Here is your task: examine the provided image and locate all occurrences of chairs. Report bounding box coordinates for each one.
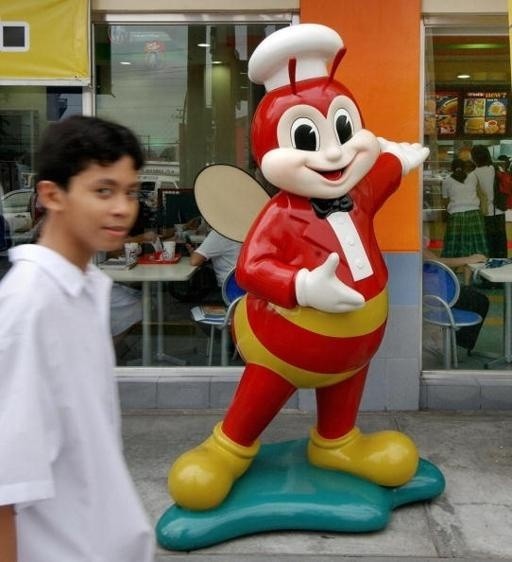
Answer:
[191,266,247,366]
[422,258,482,370]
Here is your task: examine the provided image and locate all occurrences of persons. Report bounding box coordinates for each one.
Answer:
[421,145,512,368]
[0,112,156,562]
[0,168,245,366]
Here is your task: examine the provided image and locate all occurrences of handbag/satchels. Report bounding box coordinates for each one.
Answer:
[494,169,512,211]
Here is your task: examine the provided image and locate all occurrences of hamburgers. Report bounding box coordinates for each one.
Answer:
[467,118,484,134]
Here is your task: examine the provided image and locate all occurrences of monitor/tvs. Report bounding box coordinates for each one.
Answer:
[157,187,202,235]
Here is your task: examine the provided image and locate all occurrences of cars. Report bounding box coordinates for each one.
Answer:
[0,159,181,247]
[422,162,451,207]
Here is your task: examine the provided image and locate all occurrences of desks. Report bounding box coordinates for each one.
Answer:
[99,233,203,367]
[466,257,512,370]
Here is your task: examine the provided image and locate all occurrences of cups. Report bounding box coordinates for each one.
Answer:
[124,242,138,265]
[163,241,176,259]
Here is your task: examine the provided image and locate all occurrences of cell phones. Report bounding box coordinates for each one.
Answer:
[185,235,192,247]
